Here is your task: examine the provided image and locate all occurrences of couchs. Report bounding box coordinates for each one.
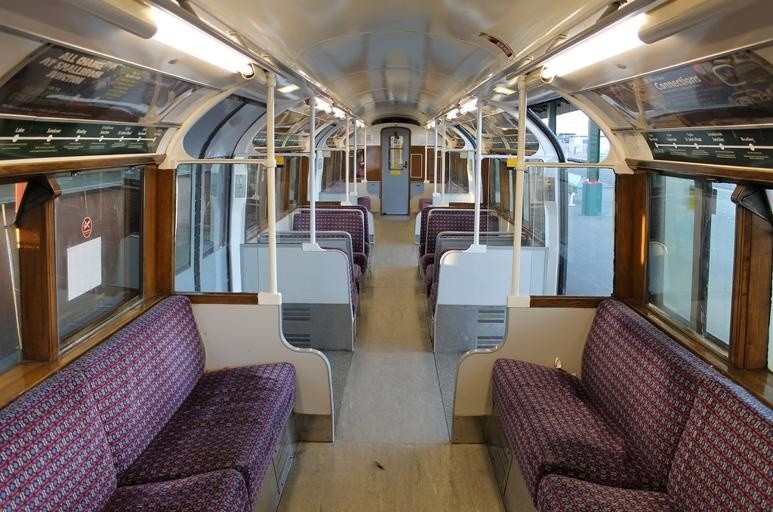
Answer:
[535,374,773,512]
[258,194,370,314]
[486,298,721,504]
[420,201,528,327]
[0,363,250,512]
[69,296,300,512]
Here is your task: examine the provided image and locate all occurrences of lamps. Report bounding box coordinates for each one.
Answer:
[539,7,653,82]
[146,6,253,80]
